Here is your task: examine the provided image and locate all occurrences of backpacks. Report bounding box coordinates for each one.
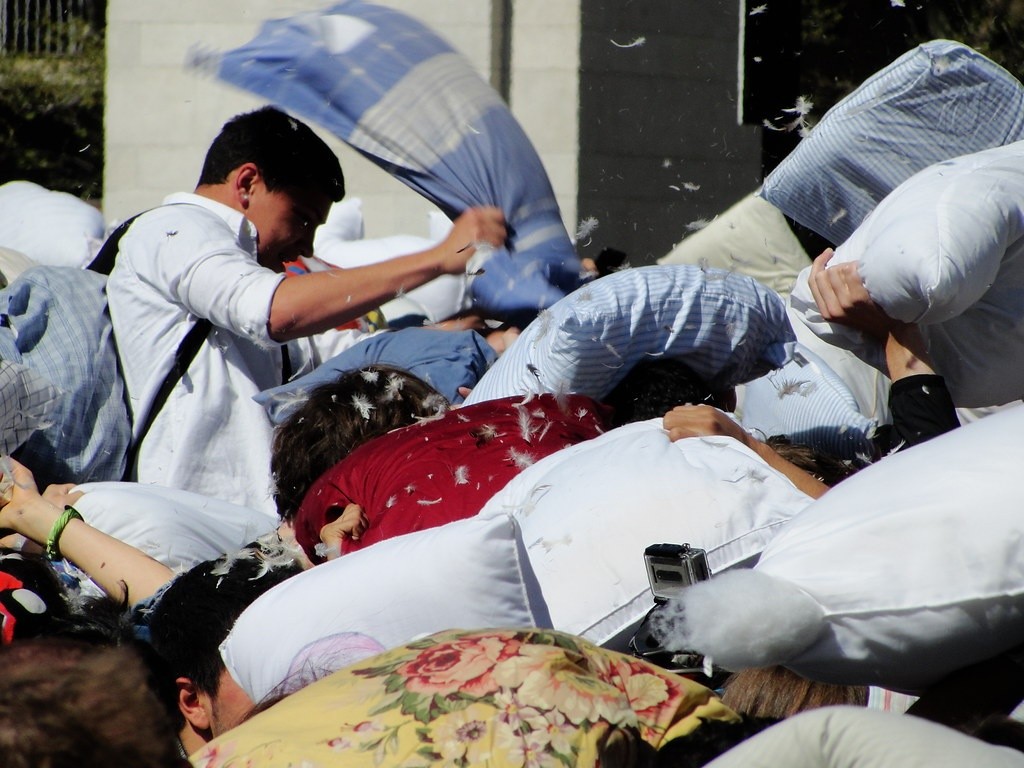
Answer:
[0,204,218,495]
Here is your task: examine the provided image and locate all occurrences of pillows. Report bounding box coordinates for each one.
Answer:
[0,265,134,495]
[743,341,879,461]
[251,327,500,431]
[187,628,743,768]
[0,359,72,456]
[652,402,1024,688]
[656,193,814,303]
[0,248,35,291]
[461,264,798,408]
[69,481,280,597]
[314,198,473,325]
[698,704,1024,768]
[755,39,1024,245]
[0,180,106,286]
[477,405,815,651]
[216,512,552,707]
[736,304,894,424]
[787,142,1024,407]
[185,0,587,312]
[293,393,613,566]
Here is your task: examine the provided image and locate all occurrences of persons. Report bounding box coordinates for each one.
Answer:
[2,206,1024,768]
[102,100,509,524]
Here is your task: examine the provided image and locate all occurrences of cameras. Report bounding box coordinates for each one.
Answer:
[627,543,713,678]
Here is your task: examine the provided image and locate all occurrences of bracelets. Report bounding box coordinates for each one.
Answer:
[46,503,85,561]
